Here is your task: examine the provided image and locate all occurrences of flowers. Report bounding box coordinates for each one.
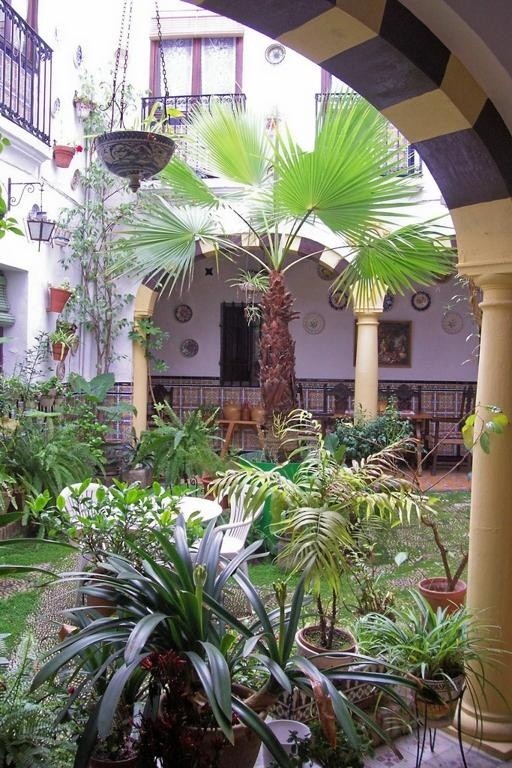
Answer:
[76,96,98,109]
[73,142,83,152]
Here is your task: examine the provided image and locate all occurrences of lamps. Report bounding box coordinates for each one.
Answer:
[7,176,55,252]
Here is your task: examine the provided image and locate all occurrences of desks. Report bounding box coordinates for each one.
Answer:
[121,494,223,541]
[300,413,433,477]
[216,418,265,459]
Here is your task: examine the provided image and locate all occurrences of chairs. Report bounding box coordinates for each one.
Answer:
[384,384,428,466]
[295,383,307,429]
[420,385,475,478]
[189,484,266,613]
[59,481,138,551]
[322,384,354,442]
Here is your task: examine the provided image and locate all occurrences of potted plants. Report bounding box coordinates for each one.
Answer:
[49,281,73,314]
[80,102,185,192]
[1,368,151,517]
[50,331,77,359]
[353,398,509,623]
[17,504,511,768]
[197,402,218,428]
[205,403,447,679]
[94,117,462,554]
[222,266,272,325]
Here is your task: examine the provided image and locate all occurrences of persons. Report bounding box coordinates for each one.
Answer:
[379,328,408,355]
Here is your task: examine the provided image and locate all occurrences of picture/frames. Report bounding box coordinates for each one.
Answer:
[350,317,414,370]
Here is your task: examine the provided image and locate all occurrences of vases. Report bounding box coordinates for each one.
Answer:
[252,400,268,424]
[204,477,228,507]
[241,401,251,421]
[53,145,73,167]
[76,107,91,118]
[221,398,241,421]
